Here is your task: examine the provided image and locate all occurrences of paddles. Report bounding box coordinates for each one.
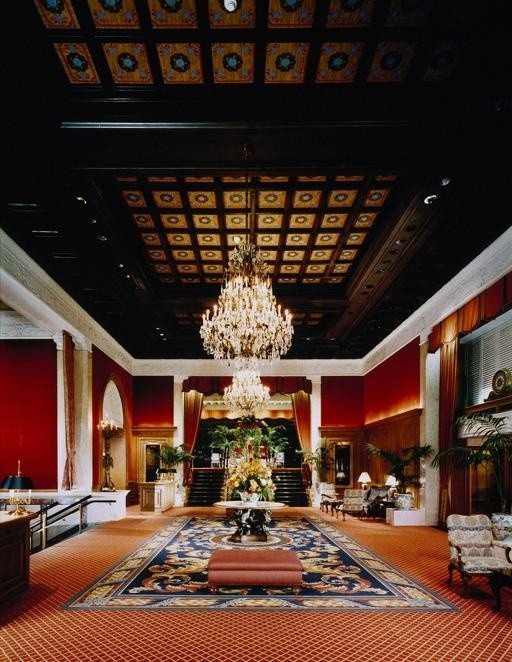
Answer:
[59,514,462,612]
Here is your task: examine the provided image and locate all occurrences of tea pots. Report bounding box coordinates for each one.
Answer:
[332,441,353,488]
[144,443,162,482]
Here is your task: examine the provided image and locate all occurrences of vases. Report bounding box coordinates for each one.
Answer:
[226,457,277,501]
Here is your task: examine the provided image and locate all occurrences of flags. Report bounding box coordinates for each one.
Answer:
[236,490,260,502]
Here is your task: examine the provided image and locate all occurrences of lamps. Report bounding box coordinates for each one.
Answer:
[446,513,512,610]
[318,482,399,521]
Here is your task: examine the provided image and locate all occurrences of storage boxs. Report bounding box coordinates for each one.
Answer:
[207,550,304,595]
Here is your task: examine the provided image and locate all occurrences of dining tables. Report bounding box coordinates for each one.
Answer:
[360,439,435,510]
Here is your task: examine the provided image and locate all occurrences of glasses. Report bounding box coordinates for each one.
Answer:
[97,413,118,492]
[336,472,346,481]
[224,369,270,420]
[1,476,36,517]
[358,471,371,490]
[156,468,177,483]
[384,475,400,499]
[198,239,296,368]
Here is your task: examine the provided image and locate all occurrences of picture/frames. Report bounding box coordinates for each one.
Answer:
[213,500,288,543]
